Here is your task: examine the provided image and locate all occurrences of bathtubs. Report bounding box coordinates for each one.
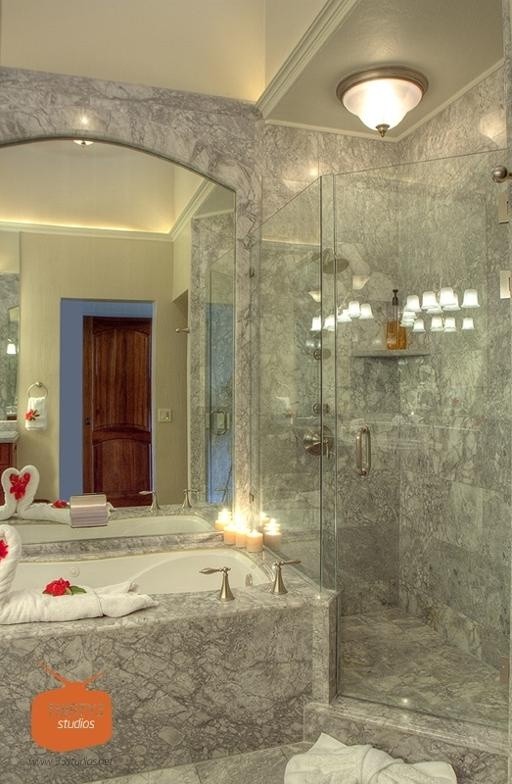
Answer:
[0,514,217,544]
[10,546,274,595]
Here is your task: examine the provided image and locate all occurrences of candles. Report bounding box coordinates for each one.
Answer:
[223,524,264,553]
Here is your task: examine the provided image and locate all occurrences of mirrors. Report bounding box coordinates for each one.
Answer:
[0,135,236,546]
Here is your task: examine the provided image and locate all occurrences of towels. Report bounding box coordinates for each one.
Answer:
[0,523,159,626]
[282,733,458,784]
[70,493,109,530]
[24,396,48,430]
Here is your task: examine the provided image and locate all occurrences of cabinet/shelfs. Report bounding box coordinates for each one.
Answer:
[0,442,19,507]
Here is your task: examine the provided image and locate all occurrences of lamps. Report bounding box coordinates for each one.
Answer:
[311,286,481,340]
[337,67,428,139]
